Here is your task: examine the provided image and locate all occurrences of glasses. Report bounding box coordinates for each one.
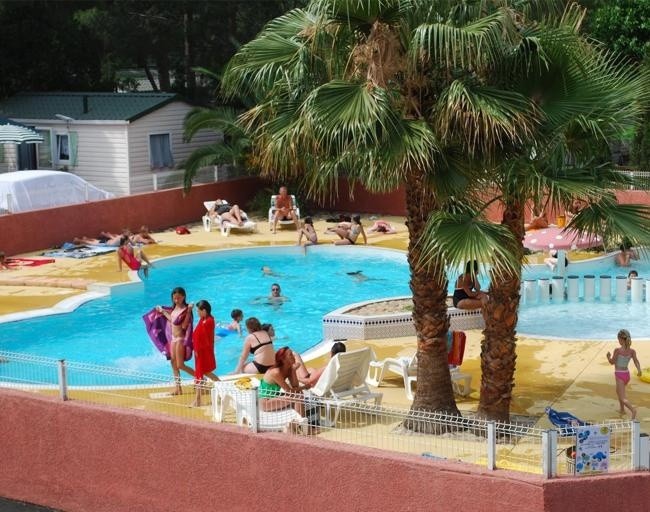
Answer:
[272,289,278,291]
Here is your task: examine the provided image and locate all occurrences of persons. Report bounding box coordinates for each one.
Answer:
[210,199,244,228]
[141,284,353,436]
[452,259,496,328]
[615,239,639,296]
[272,186,299,233]
[545,242,569,274]
[326,213,391,245]
[602,328,644,423]
[296,216,317,250]
[69,225,154,271]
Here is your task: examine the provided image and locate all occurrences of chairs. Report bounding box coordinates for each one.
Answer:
[210,327,472,434]
[202,200,257,239]
[267,195,300,232]
